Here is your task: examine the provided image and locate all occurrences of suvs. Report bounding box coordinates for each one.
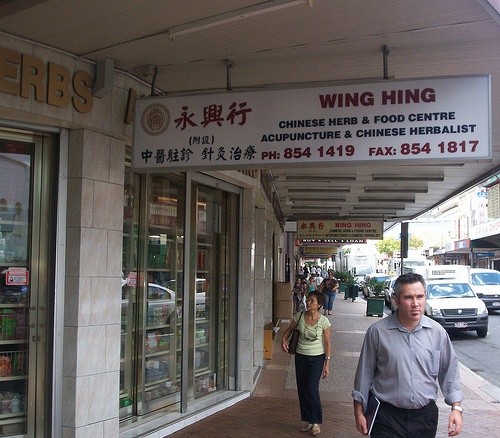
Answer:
[384,276,399,310]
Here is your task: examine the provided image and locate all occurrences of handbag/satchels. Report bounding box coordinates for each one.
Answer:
[289,310,305,354]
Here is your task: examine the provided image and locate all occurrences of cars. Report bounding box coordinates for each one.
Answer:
[162,278,207,314]
[121,280,177,327]
[363,274,391,299]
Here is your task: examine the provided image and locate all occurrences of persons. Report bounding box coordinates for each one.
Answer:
[291,263,339,314]
[352,273,465,438]
[281,291,331,436]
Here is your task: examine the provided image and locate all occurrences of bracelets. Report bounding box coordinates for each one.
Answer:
[324,356,331,360]
[452,406,464,414]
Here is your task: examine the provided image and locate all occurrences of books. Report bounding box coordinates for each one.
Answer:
[364,391,381,437]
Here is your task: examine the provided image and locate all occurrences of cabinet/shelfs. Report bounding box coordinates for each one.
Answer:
[120,218,228,399]
[0,203,28,426]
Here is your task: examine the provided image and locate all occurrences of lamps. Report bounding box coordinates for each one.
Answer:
[283,173,445,217]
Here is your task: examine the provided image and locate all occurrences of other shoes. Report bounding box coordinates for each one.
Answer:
[328,311,332,315]
[312,424,321,436]
[324,312,327,315]
[300,422,310,432]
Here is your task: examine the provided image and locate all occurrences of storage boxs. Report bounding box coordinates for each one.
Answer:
[264,329,277,359]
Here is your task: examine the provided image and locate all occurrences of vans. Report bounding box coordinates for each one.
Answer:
[469,268,500,311]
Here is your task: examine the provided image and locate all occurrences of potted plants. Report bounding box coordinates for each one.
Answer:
[334,271,359,298]
[364,276,389,317]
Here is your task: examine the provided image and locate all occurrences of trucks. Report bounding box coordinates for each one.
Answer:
[335,244,377,291]
[392,250,426,276]
[402,265,488,339]
